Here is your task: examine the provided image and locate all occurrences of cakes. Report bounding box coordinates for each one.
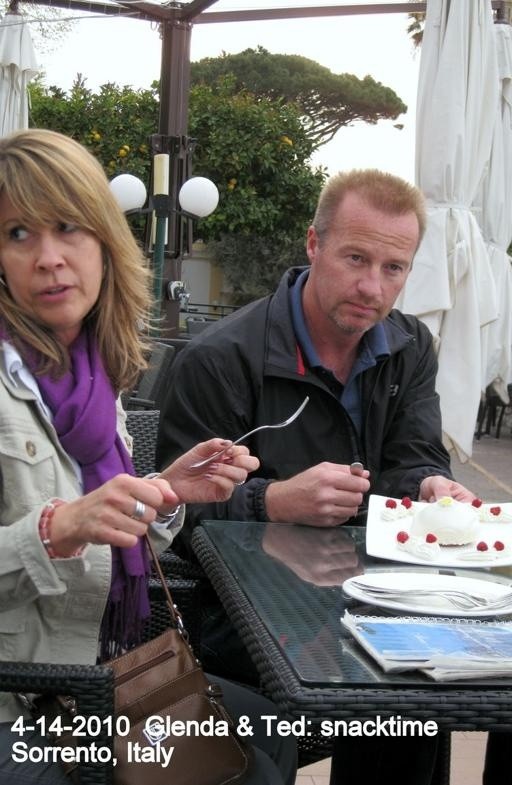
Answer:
[381,494,512,563]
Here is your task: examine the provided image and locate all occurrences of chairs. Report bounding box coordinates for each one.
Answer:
[129,314,230,407]
[474,382,512,440]
[125,408,206,578]
[0,574,206,785]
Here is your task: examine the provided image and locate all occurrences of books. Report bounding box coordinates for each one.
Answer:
[340,613,511,683]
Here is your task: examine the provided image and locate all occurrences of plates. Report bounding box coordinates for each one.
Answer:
[341,573,512,615]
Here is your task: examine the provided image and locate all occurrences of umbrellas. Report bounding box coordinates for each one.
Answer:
[0,14,40,138]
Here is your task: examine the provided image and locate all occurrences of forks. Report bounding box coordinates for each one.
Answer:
[188,395,310,470]
[350,580,512,611]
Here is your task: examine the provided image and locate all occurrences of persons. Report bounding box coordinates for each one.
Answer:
[0,129,298,785]
[155,169,512,785]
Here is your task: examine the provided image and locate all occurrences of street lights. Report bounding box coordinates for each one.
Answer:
[107,152,221,341]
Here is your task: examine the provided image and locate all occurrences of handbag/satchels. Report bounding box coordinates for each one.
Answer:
[30,626,254,785]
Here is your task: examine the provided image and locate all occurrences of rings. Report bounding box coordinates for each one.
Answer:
[130,499,146,521]
[233,480,246,486]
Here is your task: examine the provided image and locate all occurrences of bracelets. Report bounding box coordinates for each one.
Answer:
[143,472,181,524]
[39,499,87,558]
